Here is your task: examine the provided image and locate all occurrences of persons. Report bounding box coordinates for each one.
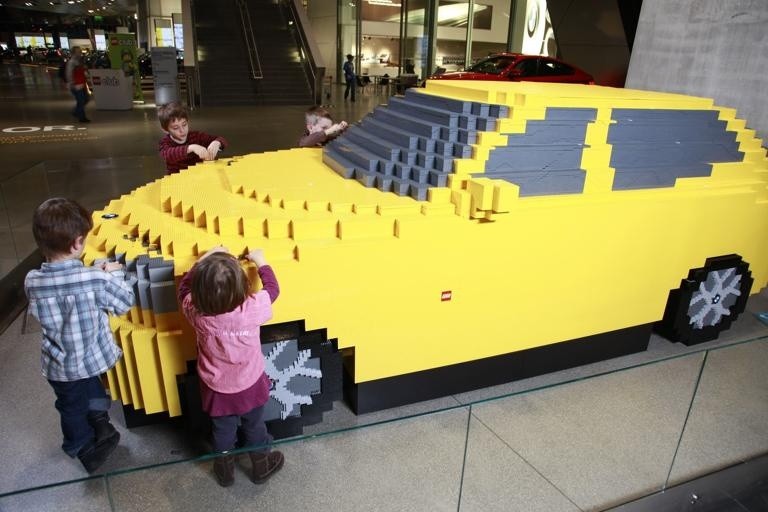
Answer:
[23,196,136,476]
[156,100,229,175]
[342,53,357,102]
[176,246,286,488]
[295,106,350,149]
[405,58,416,74]
[0,43,109,95]
[64,45,91,123]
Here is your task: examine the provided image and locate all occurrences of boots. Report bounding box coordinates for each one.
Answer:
[247,442,285,485]
[210,451,236,486]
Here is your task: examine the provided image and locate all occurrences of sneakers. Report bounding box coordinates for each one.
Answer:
[77,425,122,474]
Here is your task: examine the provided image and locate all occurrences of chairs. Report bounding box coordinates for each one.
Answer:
[356,72,390,96]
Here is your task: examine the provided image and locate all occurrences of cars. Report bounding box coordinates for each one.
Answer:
[17,44,183,74]
[422,50,593,89]
